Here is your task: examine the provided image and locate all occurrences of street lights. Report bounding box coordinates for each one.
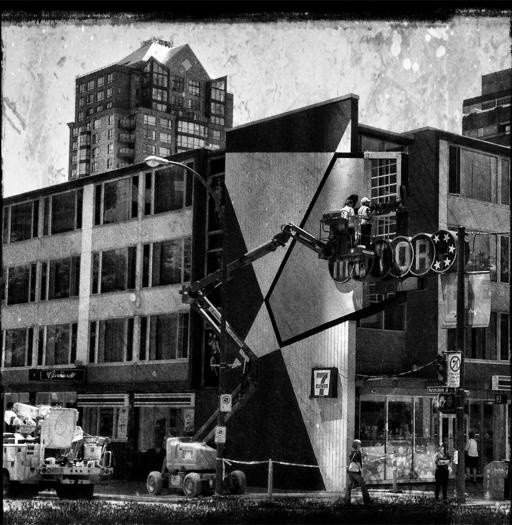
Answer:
[143,155,228,499]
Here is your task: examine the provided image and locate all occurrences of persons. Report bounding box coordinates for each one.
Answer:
[452,448,458,476]
[344,438,375,507]
[433,444,454,506]
[340,198,357,246]
[358,196,378,244]
[464,431,479,484]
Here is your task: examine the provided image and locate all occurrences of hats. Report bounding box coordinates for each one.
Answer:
[361,197,371,204]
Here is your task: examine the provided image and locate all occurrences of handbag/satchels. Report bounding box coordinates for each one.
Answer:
[349,452,360,472]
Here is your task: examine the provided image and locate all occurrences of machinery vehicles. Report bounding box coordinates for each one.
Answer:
[144,435,248,499]
[2,401,115,501]
